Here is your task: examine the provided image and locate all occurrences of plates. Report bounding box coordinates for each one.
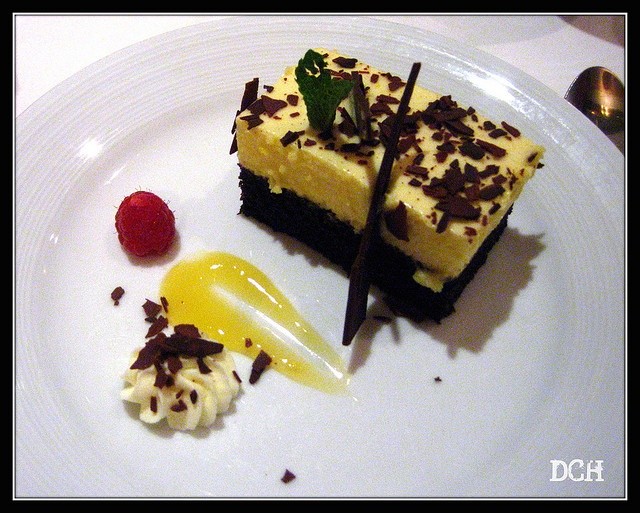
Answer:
[16,16,624,497]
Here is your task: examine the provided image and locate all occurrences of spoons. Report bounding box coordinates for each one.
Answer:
[564,66,625,155]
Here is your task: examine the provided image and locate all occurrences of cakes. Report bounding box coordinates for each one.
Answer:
[230,45,545,324]
